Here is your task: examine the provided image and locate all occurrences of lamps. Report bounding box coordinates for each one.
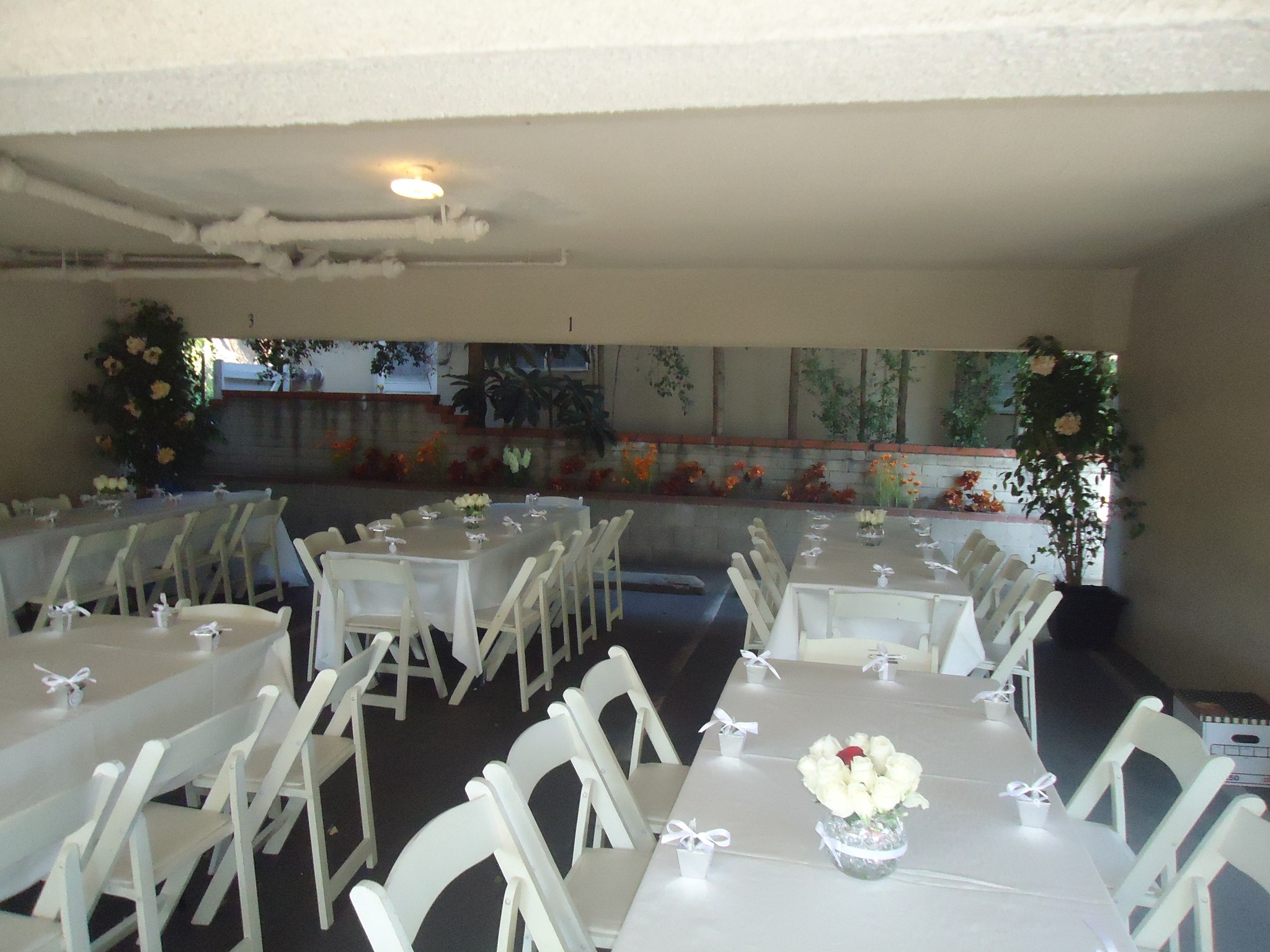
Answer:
[390,166,445,201]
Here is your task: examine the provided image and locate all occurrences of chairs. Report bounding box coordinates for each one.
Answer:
[0,493,693,952]
[726,521,1269,952]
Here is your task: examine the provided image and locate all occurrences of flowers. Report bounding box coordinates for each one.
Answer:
[936,469,1006,513]
[453,493,492,509]
[66,298,227,499]
[991,328,1151,588]
[312,429,921,510]
[792,728,928,816]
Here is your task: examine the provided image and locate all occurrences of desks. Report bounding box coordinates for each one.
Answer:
[0,490,310,611]
[608,653,1140,951]
[762,512,986,677]
[1,615,302,910]
[313,502,590,695]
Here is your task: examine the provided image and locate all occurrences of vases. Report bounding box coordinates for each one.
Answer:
[1044,584,1126,655]
[818,806,909,877]
[460,507,486,528]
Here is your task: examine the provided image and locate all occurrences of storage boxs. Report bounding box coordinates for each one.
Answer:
[1170,690,1270,788]
